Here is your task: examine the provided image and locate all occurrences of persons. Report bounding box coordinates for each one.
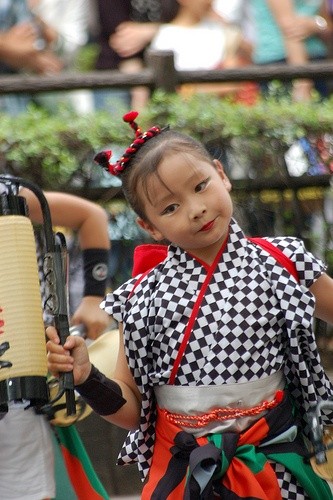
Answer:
[0,110,333,500]
[0,0,333,115]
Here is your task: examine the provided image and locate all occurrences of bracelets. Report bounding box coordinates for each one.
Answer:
[314,15,328,30]
